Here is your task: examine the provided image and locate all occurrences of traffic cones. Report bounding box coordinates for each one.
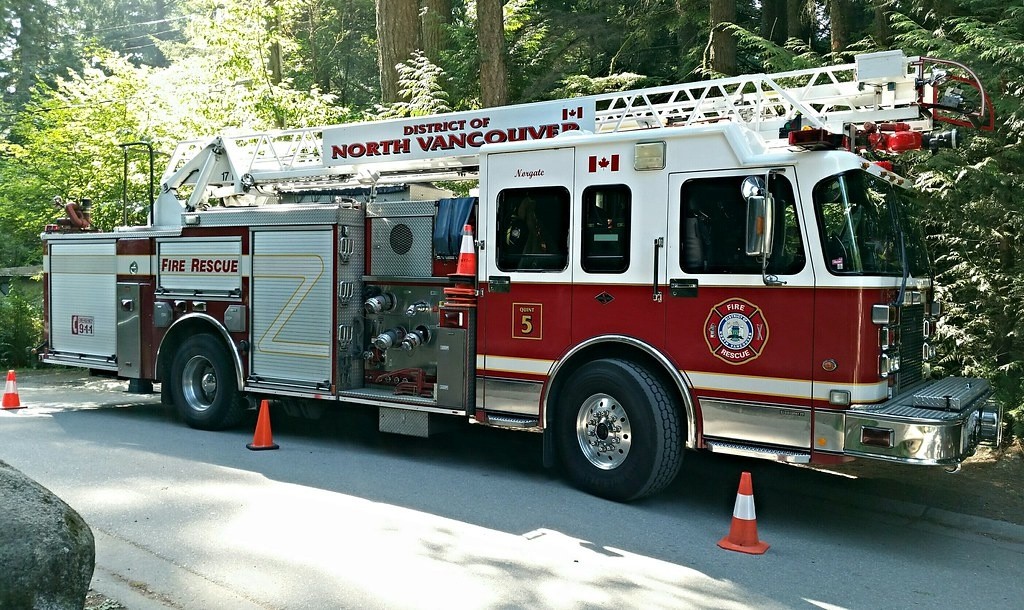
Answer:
[443,223,477,308]
[716,472,771,555]
[246,400,280,451]
[0,370,28,410]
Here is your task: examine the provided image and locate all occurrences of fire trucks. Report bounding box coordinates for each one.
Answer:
[31,49,1014,504]
